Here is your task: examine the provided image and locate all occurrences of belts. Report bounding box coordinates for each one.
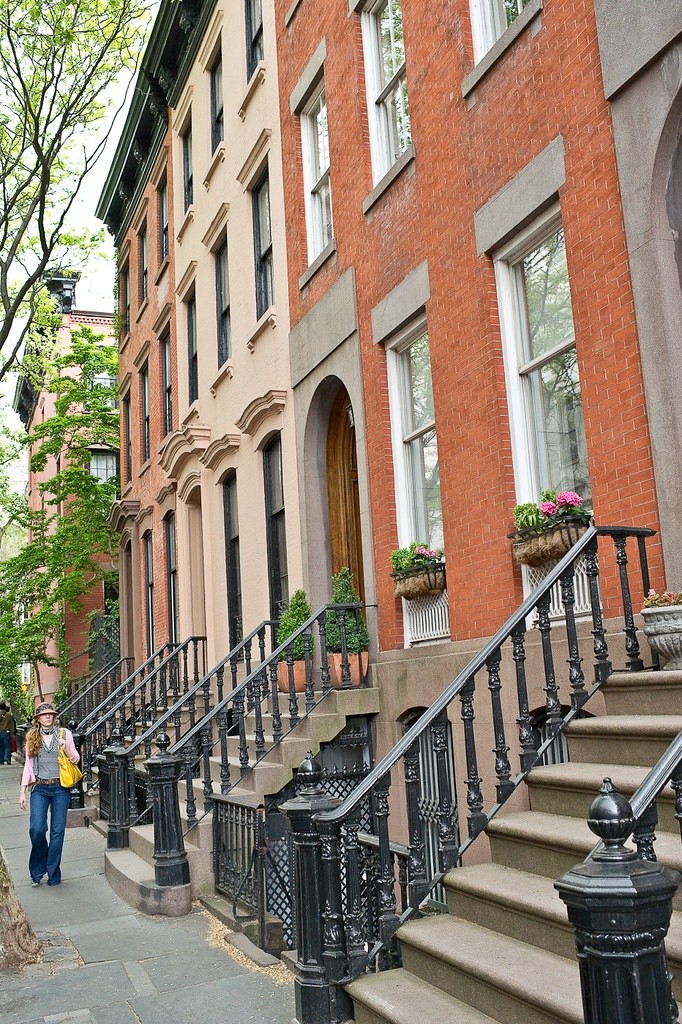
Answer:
[35,779,60,785]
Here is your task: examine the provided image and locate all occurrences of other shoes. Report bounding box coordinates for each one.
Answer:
[30,877,39,887]
[7,761,11,765]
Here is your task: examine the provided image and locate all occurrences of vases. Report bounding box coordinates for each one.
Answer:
[640,604,682,669]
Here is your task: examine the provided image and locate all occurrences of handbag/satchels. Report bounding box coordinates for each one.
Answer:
[58,728,82,788]
[10,736,17,752]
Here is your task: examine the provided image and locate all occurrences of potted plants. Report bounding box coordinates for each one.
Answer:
[276,590,314,692]
[323,567,369,688]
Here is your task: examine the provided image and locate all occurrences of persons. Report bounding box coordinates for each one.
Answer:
[0,701,17,765]
[19,701,80,887]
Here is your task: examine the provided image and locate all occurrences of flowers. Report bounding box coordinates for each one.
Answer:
[389,544,443,570]
[513,489,589,535]
[643,588,682,607]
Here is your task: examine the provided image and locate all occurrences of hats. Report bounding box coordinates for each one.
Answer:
[32,702,57,719]
